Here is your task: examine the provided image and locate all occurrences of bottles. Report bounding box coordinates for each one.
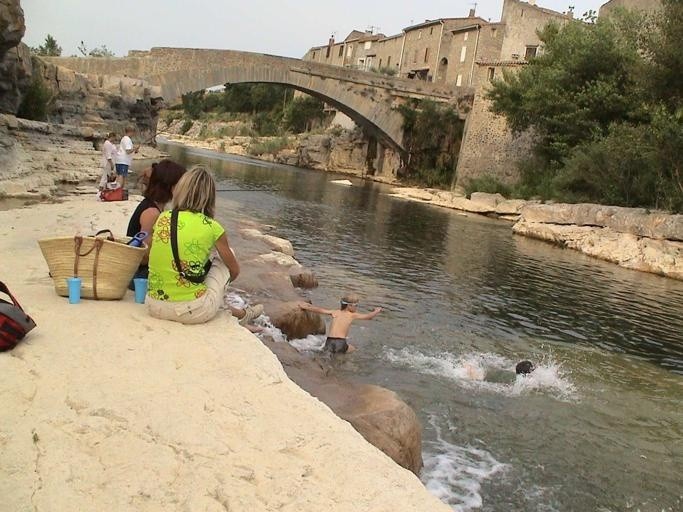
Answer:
[127,231,147,248]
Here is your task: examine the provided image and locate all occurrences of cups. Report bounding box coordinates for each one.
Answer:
[133,278,147,304]
[67,279,81,303]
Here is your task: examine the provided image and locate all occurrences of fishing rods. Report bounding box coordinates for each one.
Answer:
[135,115,188,153]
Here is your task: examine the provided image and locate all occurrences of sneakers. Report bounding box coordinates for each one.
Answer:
[239,304,264,327]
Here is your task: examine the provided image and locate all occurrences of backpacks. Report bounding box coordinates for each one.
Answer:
[0,281,36,352]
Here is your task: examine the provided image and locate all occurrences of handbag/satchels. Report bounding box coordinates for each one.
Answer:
[100,171,128,201]
[38,235,148,301]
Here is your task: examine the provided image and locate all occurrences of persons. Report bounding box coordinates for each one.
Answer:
[145,163,264,324]
[99,132,117,199]
[516,361,535,374]
[126,160,186,290]
[297,295,382,354]
[133,162,158,192]
[115,126,140,187]
